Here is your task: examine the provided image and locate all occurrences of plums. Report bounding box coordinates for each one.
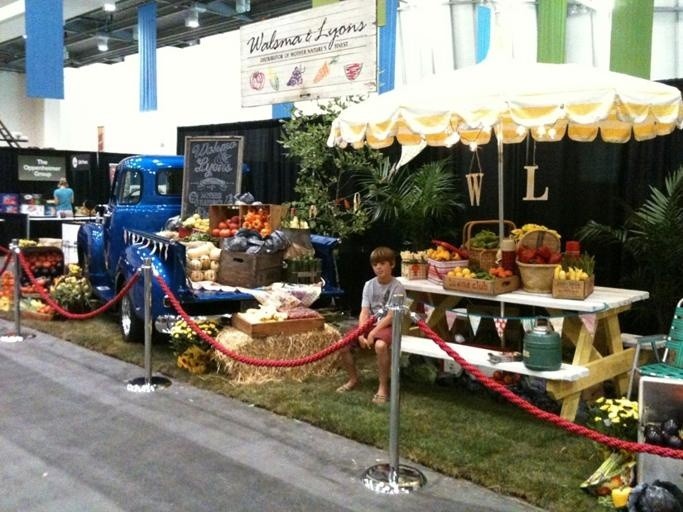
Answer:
[20,251,64,288]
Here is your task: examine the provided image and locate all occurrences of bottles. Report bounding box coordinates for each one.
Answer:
[500,239,516,275]
[565,240,580,259]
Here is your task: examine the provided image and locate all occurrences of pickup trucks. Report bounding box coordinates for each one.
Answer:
[74,153,345,343]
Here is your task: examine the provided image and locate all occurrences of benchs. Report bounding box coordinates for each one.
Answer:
[393,331,590,391]
[617,327,670,353]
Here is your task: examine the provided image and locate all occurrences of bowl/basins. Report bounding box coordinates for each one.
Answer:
[579,460,637,498]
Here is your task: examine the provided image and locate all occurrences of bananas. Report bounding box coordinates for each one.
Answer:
[554,266,589,282]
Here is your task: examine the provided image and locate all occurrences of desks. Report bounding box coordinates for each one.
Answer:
[390,266,653,428]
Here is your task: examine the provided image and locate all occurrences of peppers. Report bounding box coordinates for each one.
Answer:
[427,245,462,262]
[470,229,499,249]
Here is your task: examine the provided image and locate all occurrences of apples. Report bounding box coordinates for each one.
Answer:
[446,266,476,279]
[212,207,272,239]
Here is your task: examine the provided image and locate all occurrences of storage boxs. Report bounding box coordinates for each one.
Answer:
[438,270,520,298]
[213,243,284,292]
[549,267,594,301]
[419,238,472,285]
[205,203,239,240]
[239,204,283,237]
[231,312,325,338]
[399,262,427,281]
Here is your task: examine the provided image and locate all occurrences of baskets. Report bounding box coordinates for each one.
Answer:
[515,230,561,294]
[461,219,517,271]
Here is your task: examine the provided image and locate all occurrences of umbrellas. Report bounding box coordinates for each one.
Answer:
[326,56,683,249]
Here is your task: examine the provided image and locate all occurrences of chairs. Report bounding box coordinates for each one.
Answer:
[624,294,681,408]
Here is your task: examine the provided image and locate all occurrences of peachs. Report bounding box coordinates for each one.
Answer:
[488,267,512,280]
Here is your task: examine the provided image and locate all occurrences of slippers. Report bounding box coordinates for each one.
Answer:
[335,382,361,393]
[372,389,388,405]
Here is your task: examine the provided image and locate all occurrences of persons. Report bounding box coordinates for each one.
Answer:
[45,176,74,218]
[23,194,36,211]
[333,244,406,404]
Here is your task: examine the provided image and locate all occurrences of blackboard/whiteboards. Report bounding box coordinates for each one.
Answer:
[181,135,244,222]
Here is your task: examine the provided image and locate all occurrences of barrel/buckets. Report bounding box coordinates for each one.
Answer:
[282,256,323,284]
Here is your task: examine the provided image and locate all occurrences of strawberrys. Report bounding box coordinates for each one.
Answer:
[519,245,561,265]
